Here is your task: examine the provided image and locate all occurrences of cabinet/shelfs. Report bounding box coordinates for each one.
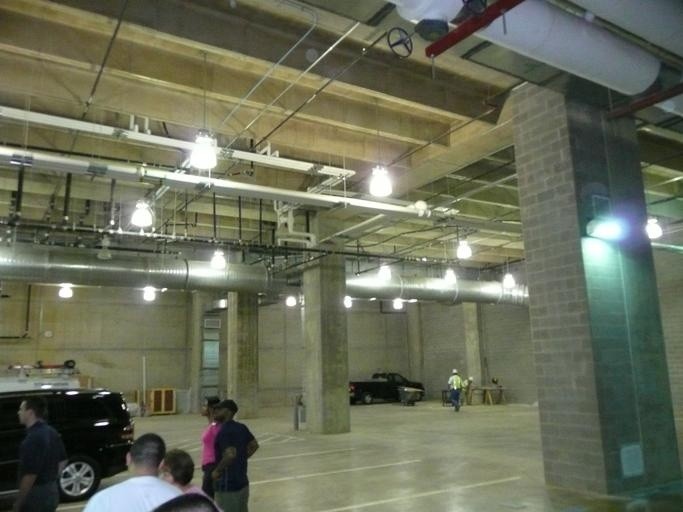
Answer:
[150,388,177,416]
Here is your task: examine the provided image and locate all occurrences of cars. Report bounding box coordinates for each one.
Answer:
[0,363,136,503]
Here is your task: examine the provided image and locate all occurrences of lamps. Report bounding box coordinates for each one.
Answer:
[368,96,393,197]
[188,50,218,171]
[456,224,515,289]
[131,182,155,228]
[642,163,664,238]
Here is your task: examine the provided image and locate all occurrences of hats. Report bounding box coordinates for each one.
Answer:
[211,398,239,414]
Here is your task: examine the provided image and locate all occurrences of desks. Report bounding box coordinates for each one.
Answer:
[466,385,510,408]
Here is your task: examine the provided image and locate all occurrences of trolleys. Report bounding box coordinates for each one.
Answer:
[391,385,422,405]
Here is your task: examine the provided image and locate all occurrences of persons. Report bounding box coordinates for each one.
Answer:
[152,492,221,511]
[10,393,70,511]
[447,368,463,412]
[211,398,260,511]
[158,448,226,511]
[81,432,185,511]
[461,376,474,405]
[198,395,221,498]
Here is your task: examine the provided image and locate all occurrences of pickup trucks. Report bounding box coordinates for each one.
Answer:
[349,371,425,404]
